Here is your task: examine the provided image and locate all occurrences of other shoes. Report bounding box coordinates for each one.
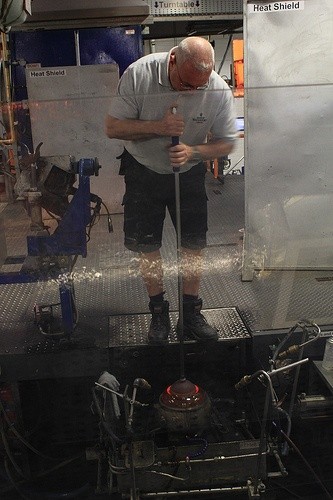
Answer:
[176,298,219,344]
[147,300,171,342]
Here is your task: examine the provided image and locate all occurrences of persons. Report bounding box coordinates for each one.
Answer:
[107,37,236,343]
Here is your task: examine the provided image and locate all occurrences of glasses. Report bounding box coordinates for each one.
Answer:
[175,58,209,90]
